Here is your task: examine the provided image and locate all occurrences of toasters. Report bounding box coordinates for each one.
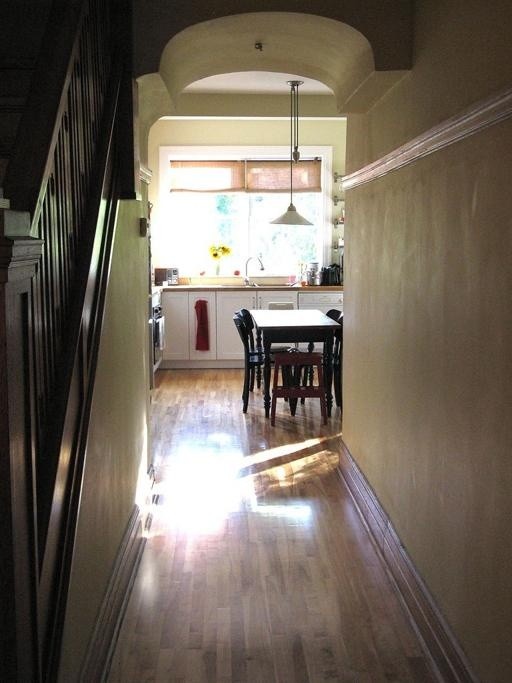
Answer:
[155,268,178,286]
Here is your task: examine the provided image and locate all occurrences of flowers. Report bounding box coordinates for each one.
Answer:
[208,245,231,260]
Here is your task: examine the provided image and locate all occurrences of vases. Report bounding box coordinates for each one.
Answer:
[215,263,221,276]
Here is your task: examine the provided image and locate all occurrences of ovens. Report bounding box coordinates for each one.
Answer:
[153,304,165,365]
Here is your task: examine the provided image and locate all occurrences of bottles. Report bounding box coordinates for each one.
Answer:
[298,263,318,285]
[312,275,320,285]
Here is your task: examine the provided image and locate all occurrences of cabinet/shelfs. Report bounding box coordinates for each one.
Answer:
[216,291,297,361]
[299,293,343,366]
[161,291,217,361]
[333,172,344,252]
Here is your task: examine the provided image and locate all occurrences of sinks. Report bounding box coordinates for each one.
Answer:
[222,283,256,287]
[258,282,295,287]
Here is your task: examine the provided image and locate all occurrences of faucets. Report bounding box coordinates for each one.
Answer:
[245,254,264,285]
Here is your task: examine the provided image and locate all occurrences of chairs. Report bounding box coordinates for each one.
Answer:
[233,302,343,427]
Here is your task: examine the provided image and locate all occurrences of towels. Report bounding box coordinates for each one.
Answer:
[194,300,209,350]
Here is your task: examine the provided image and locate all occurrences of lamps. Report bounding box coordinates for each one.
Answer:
[269,81,315,226]
